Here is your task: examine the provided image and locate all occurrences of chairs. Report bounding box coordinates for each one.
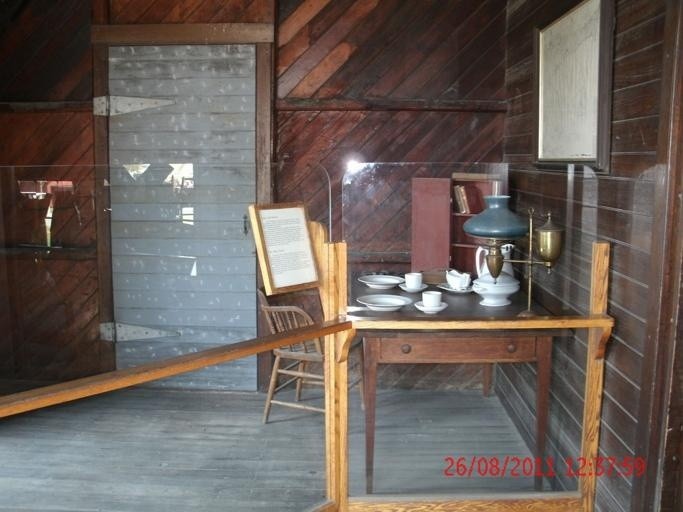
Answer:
[256,287,326,425]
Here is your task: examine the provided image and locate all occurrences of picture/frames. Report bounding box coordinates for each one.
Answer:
[531,1,616,176]
[247,201,319,298]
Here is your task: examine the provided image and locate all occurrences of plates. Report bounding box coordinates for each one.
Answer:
[357,271,477,313]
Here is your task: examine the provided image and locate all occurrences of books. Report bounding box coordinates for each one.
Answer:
[454,185,471,214]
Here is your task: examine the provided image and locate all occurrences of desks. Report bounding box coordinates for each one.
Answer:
[349,263,575,496]
